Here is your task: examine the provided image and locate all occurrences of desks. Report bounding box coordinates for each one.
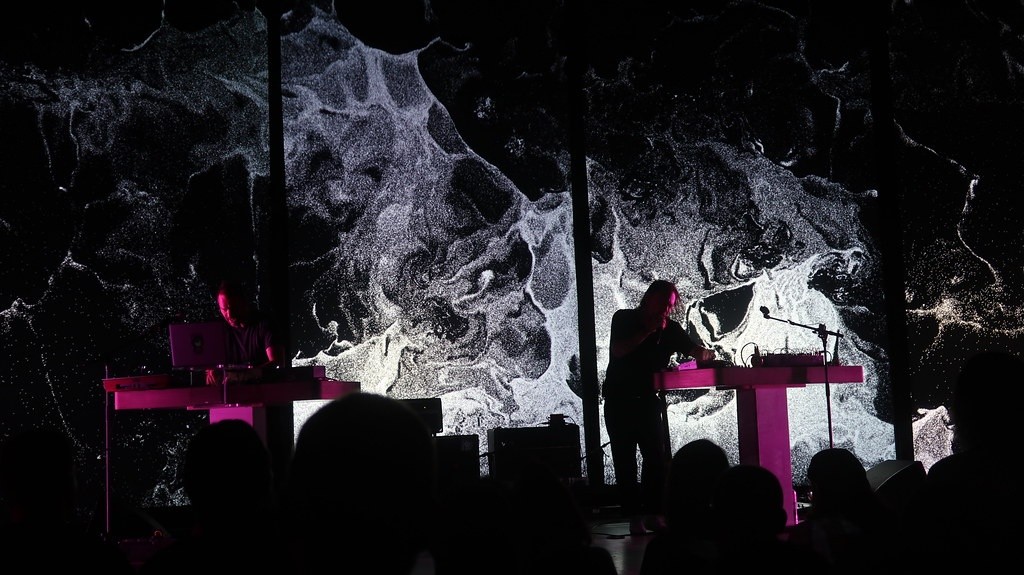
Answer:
[658,364,865,524]
[112,381,362,447]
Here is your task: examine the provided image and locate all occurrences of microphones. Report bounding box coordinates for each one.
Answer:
[656,328,663,344]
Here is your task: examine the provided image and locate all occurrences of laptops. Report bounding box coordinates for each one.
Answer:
[170,322,253,370]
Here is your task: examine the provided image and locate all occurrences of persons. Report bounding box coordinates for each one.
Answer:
[602,281,713,535]
[205,281,281,384]
[0,393,618,575]
[639,440,1024,575]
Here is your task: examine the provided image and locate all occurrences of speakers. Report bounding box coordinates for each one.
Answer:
[430,434,480,481]
[488,424,582,480]
[866,460,927,517]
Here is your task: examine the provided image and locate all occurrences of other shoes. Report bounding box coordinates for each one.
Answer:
[630,519,646,535]
